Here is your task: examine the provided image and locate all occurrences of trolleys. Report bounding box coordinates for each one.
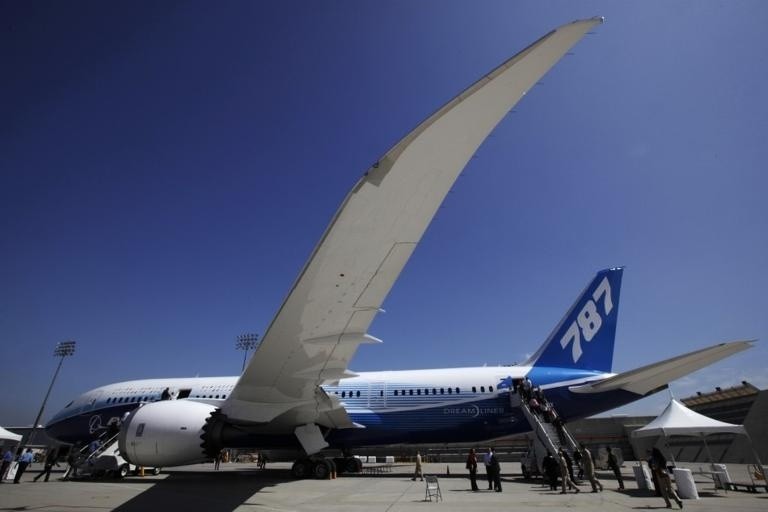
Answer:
[723,463,768,492]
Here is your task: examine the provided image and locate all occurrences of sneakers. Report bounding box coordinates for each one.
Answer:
[666,501,683,509]
[560,486,603,494]
[471,487,502,492]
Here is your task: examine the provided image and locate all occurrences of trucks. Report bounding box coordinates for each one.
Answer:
[587,447,623,470]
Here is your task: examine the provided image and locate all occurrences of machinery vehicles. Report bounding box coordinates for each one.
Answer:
[73,400,164,477]
[510,390,596,479]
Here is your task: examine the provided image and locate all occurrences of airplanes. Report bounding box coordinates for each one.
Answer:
[43,13,756,480]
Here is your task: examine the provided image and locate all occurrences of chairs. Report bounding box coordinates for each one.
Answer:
[422,473,443,504]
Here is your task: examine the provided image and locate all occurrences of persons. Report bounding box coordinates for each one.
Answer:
[648,449,683,509]
[465,446,502,492]
[215,450,225,470]
[517,378,624,494]
[33,449,61,482]
[0,445,17,484]
[64,447,84,480]
[14,447,32,484]
[258,453,269,470]
[411,450,423,481]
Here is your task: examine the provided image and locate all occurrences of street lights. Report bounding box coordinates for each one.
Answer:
[234,333,258,375]
[14,339,78,461]
